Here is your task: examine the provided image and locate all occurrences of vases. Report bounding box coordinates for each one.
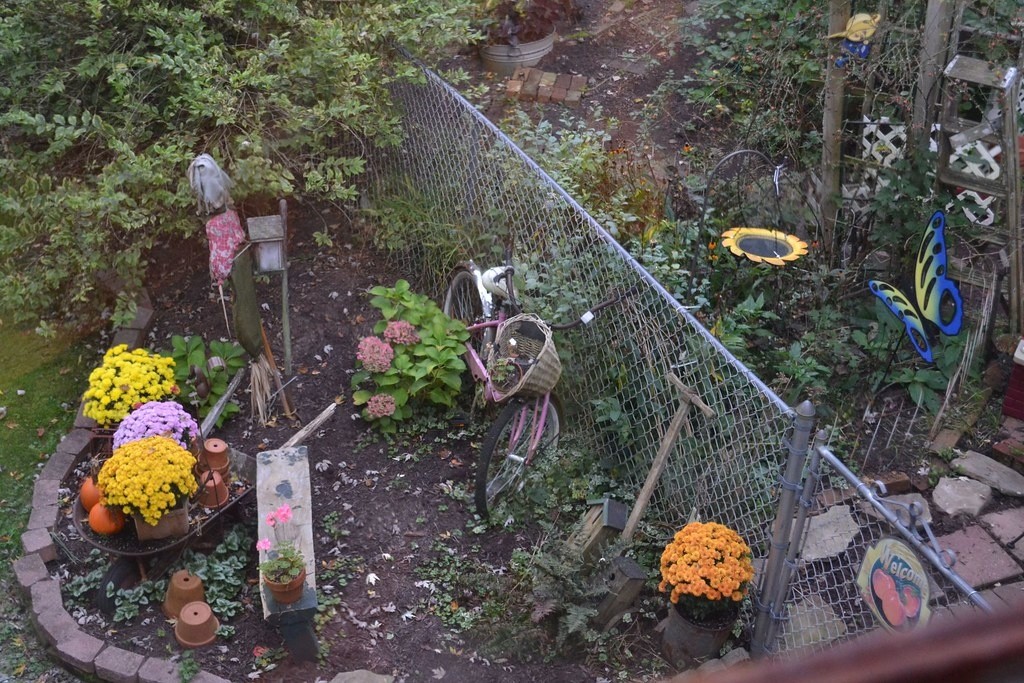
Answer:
[263,566,307,602]
[161,570,206,619]
[662,602,740,671]
[174,600,220,646]
[195,464,231,482]
[132,497,191,540]
[195,438,230,471]
[198,470,231,507]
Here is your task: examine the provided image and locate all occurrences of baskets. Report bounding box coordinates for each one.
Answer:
[486,312,562,402]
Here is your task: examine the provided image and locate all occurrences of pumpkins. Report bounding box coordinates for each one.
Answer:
[80,477,126,534]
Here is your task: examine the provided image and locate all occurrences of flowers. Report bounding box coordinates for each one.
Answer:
[658,523,754,620]
[255,503,306,584]
[98,435,199,525]
[351,278,472,435]
[113,402,201,450]
[81,344,180,428]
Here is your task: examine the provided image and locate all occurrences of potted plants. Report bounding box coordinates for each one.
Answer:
[490,358,523,393]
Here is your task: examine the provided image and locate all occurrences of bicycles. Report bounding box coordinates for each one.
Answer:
[437,222,629,525]
